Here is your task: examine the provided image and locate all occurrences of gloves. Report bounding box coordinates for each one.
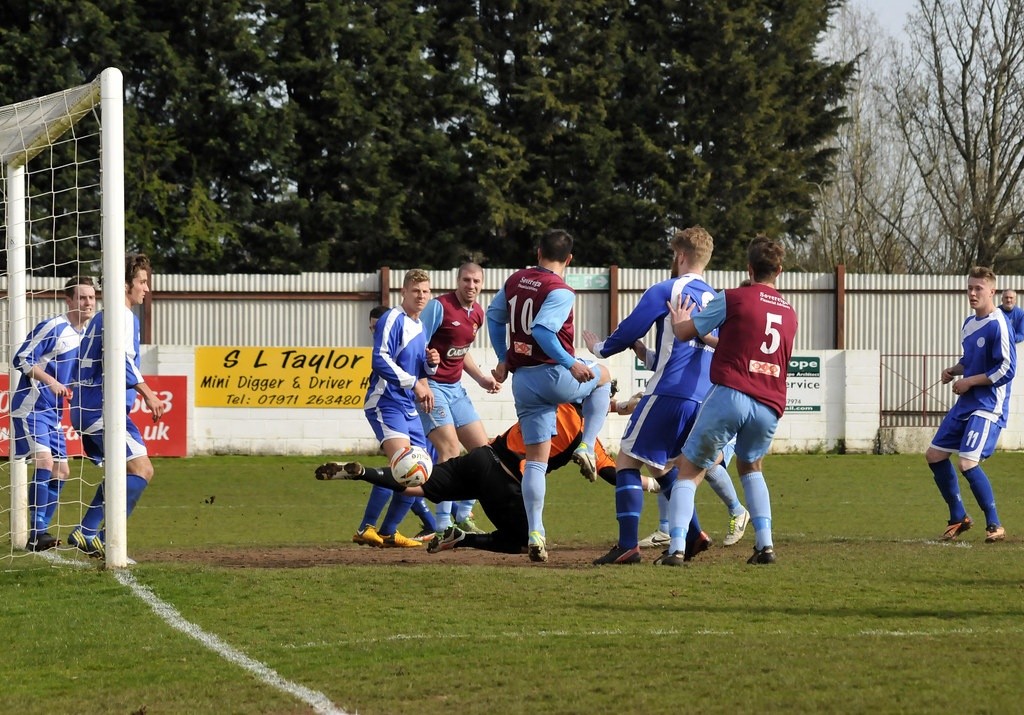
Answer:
[615,391,645,415]
[648,476,661,493]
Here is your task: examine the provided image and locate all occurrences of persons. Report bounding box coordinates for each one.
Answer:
[999,289,1024,344]
[926,269,1016,542]
[486,229,612,562]
[582,219,751,563]
[658,233,799,567]
[9,276,96,550]
[352,248,503,547]
[66,252,165,566]
[314,380,662,555]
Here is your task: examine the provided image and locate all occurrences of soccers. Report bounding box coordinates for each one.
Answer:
[391,447,433,487]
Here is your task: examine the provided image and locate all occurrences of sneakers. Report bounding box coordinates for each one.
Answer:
[942,516,973,541]
[28,535,63,552]
[686,532,712,560]
[593,545,641,564]
[377,529,422,547]
[353,524,384,548]
[529,533,549,563]
[456,518,487,535]
[316,460,366,481]
[654,550,685,568]
[411,531,436,542]
[69,526,97,554]
[427,526,465,553]
[746,546,776,565]
[572,443,597,482]
[985,523,1005,543]
[638,530,671,548]
[722,506,750,546]
[87,537,138,566]
